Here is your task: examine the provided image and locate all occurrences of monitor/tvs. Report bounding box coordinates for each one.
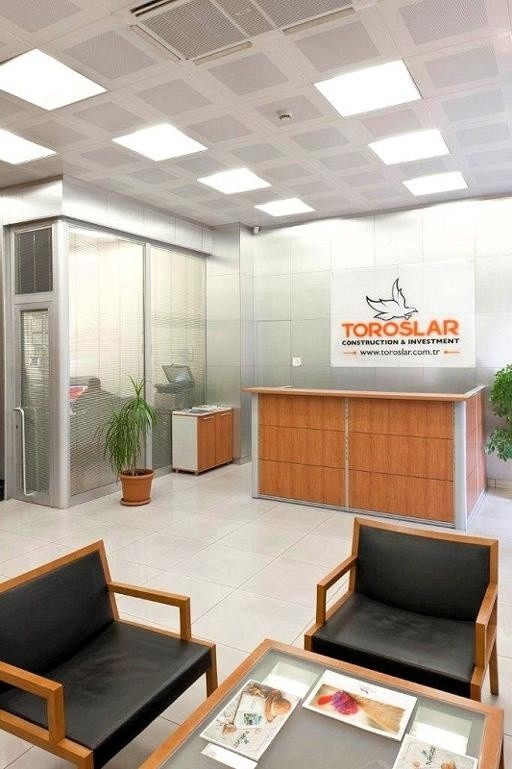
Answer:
[70,384,88,404]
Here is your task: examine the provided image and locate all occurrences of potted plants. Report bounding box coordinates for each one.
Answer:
[90,372,159,508]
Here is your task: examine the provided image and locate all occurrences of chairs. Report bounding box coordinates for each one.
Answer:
[304,517,501,703]
[0,540,217,769]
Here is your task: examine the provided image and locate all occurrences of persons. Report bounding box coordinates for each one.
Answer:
[70,376,110,414]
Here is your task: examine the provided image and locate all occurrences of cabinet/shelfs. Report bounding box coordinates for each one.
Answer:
[170,405,235,474]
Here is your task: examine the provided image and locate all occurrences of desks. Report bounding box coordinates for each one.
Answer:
[137,640,505,769]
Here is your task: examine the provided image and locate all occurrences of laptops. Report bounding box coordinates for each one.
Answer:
[162,365,195,383]
